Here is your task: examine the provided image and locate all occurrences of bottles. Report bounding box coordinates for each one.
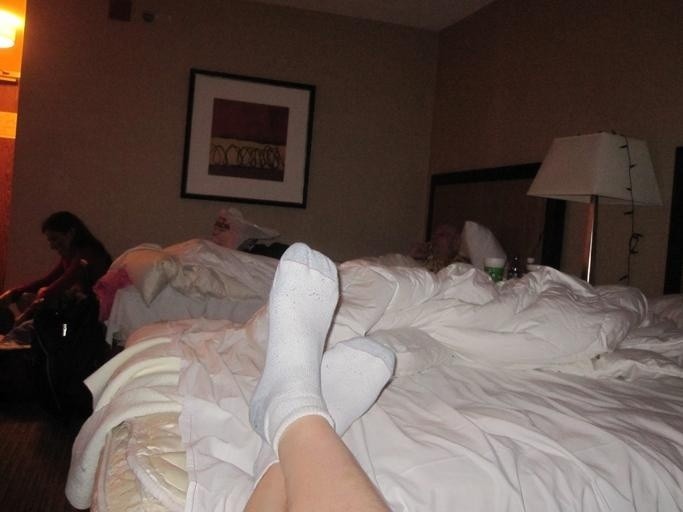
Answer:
[509,257,535,279]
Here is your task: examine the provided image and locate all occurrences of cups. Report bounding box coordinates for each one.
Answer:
[482,257,506,280]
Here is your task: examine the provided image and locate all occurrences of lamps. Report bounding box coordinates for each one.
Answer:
[521,131,661,278]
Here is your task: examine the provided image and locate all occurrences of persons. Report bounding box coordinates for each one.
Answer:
[0,208,112,404]
[236,240,400,512]
[413,224,461,271]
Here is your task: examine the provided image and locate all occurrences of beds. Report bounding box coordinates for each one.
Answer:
[100,159,562,352]
[62,139,683,512]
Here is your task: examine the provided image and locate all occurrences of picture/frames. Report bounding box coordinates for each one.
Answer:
[179,68,316,210]
